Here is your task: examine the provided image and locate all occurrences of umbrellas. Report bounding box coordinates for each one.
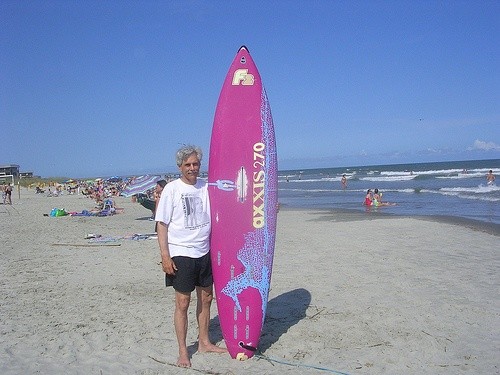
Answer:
[95,178,102,182]
[107,175,122,183]
[120,174,162,198]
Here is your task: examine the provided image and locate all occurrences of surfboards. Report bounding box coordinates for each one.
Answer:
[208,45,278,362]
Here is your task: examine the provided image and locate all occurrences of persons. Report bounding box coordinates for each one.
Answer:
[35,181,120,217]
[341,174,347,192]
[362,188,396,207]
[0,182,13,205]
[487,170,496,187]
[132,143,280,369]
